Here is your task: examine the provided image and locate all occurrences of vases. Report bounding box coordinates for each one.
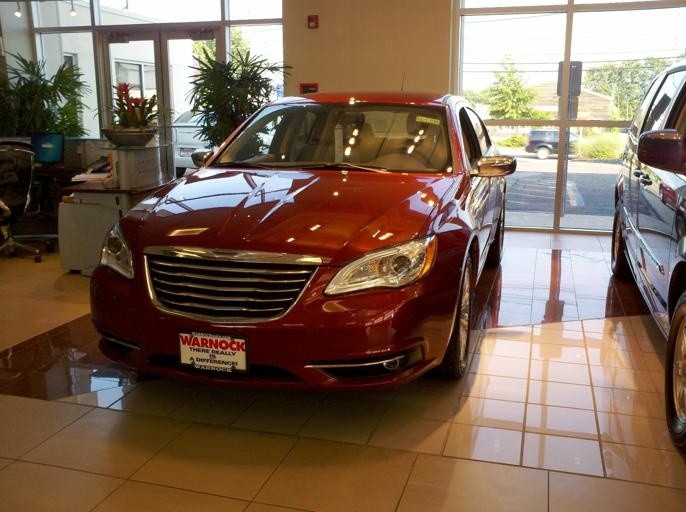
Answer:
[101,127,159,150]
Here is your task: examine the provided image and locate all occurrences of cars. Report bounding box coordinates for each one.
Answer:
[525,128,581,160]
[611,62,685,449]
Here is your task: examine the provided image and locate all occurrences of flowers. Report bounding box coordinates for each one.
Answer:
[92,83,180,127]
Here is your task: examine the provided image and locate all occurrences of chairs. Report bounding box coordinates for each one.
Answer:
[0,140,59,263]
[297,113,365,164]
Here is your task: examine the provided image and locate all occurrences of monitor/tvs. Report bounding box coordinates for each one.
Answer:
[29,133,64,171]
